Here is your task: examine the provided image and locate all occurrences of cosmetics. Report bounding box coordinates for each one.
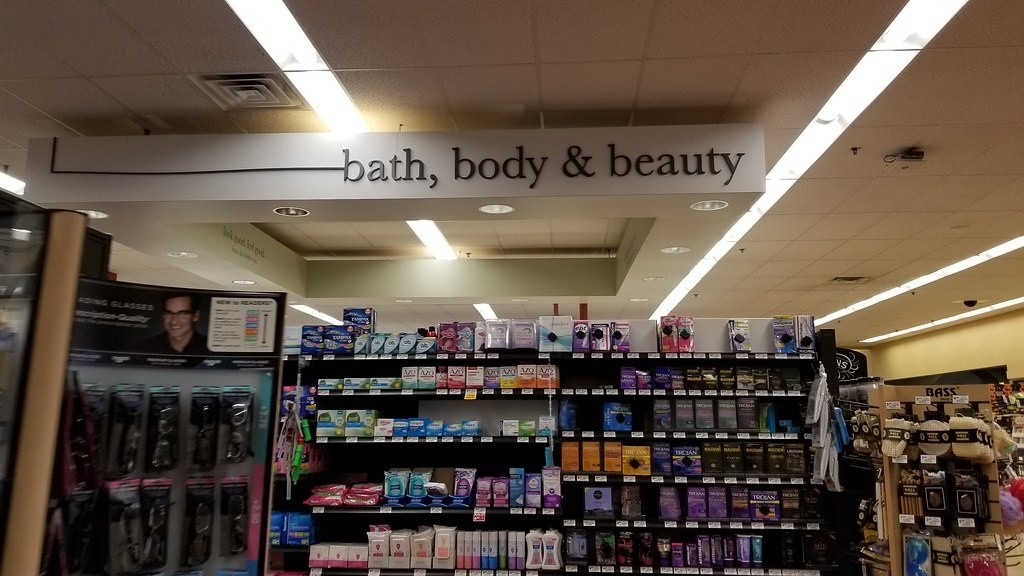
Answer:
[269,308,816,569]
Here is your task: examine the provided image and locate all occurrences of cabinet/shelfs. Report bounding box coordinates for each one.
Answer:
[265,343,561,576]
[562,315,851,576]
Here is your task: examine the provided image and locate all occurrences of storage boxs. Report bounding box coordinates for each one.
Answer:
[299,310,815,520]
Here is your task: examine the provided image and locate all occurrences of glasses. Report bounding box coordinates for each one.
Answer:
[229,494,248,554]
[123,509,141,564]
[191,402,217,465]
[186,496,213,567]
[151,404,179,470]
[161,308,195,317]
[115,408,143,473]
[225,404,249,458]
[144,504,167,565]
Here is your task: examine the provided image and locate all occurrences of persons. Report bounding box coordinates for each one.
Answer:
[154,293,208,356]
